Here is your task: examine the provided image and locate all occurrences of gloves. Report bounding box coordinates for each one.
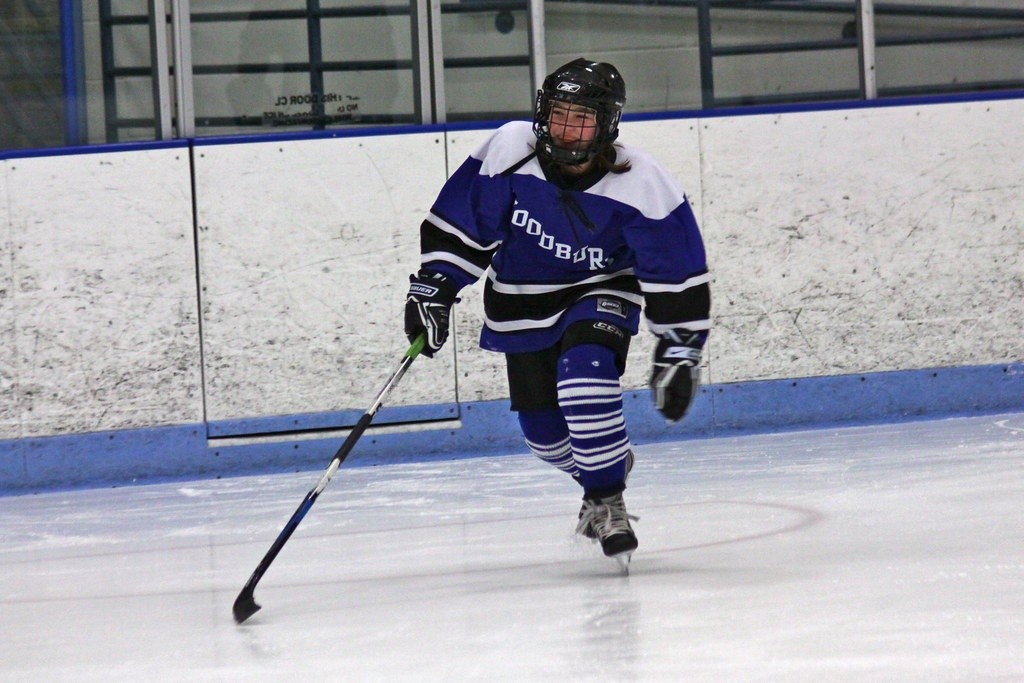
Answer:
[649,326,711,422]
[404,268,463,359]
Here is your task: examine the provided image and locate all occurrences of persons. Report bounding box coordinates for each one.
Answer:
[404,59,712,578]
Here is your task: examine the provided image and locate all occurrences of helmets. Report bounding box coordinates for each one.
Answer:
[532,58,626,167]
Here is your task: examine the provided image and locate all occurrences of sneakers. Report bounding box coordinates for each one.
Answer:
[583,493,639,575]
[576,490,597,554]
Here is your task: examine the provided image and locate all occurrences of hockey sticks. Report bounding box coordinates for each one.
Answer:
[234,334,429,624]
